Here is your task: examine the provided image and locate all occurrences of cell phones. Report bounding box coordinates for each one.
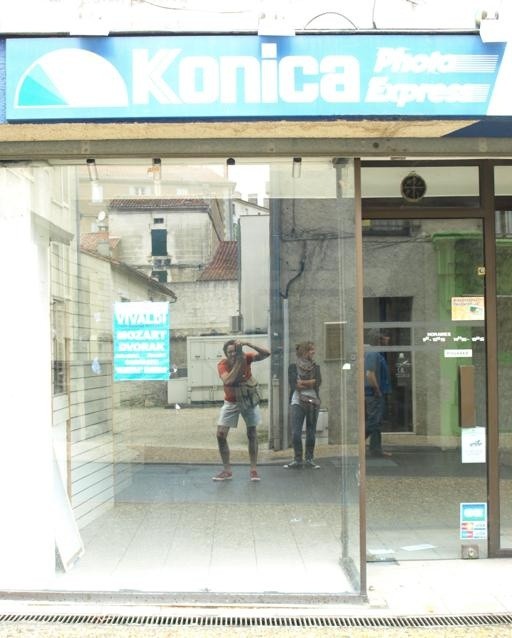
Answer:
[235,343,242,355]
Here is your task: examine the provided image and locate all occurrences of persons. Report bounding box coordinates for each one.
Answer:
[281,340,322,470]
[364,328,392,459]
[212,339,271,482]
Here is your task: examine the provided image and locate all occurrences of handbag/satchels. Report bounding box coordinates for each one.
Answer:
[236,375,261,414]
[296,393,322,413]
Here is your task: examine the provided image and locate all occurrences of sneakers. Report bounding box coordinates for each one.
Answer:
[249,470,261,481]
[304,459,321,469]
[374,451,393,457]
[283,460,304,469]
[210,470,235,481]
